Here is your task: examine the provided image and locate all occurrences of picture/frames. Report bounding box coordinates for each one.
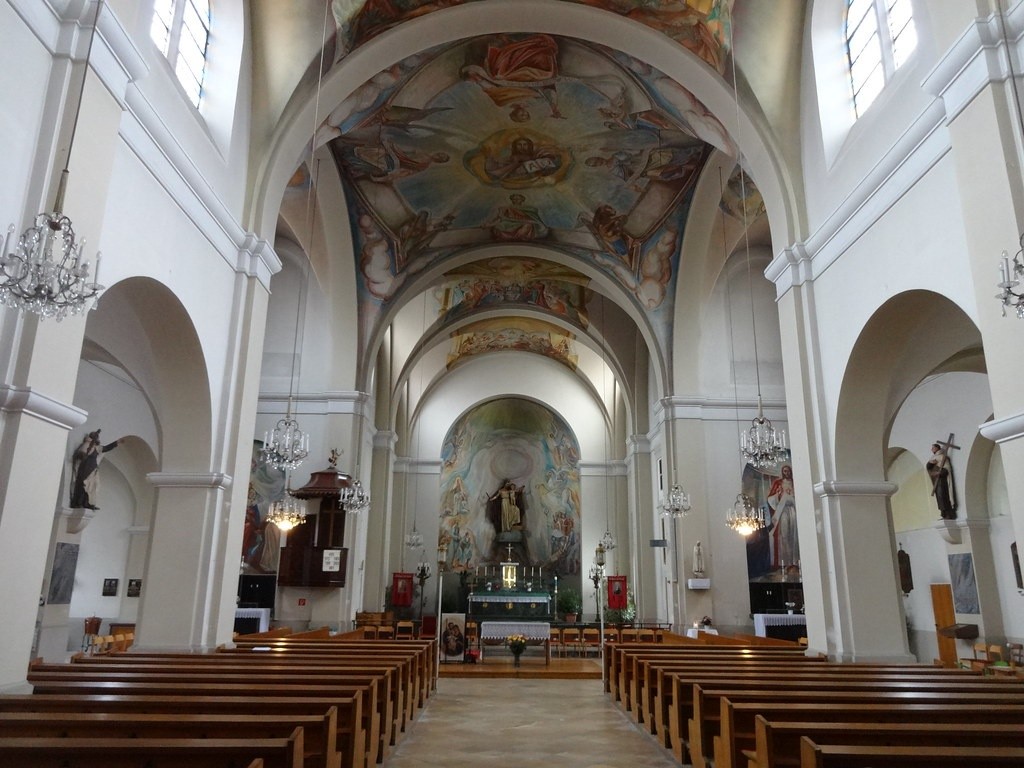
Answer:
[127,579,142,597]
[102,579,118,596]
[440,612,466,663]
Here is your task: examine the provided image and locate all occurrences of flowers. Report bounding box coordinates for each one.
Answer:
[507,634,526,646]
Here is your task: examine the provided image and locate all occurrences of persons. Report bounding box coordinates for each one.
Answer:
[69,429,125,510]
[485,479,525,532]
[926,444,957,520]
[509,484,519,505]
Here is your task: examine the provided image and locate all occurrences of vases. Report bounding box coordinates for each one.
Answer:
[510,645,524,667]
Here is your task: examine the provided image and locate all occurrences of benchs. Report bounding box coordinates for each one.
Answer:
[1,611,440,768]
[603,629,1024,768]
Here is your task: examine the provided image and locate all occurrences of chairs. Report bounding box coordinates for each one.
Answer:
[550,626,663,659]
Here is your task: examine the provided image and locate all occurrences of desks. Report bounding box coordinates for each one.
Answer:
[479,622,551,664]
[469,592,551,616]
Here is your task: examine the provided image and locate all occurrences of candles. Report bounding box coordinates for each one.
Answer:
[493,567,495,576]
[485,567,487,576]
[539,567,542,577]
[475,566,478,576]
[523,566,525,577]
[554,576,557,594]
[532,567,534,577]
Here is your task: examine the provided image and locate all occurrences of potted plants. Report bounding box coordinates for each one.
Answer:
[556,588,581,623]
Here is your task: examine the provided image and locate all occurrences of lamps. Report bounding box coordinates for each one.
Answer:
[663,218,692,517]
[405,290,426,550]
[601,294,616,552]
[589,565,604,623]
[267,160,320,530]
[435,545,447,677]
[415,561,432,628]
[1,0,104,321]
[338,480,369,515]
[726,0,791,469]
[718,167,765,535]
[994,1,1024,319]
[258,0,331,470]
[595,540,606,687]
